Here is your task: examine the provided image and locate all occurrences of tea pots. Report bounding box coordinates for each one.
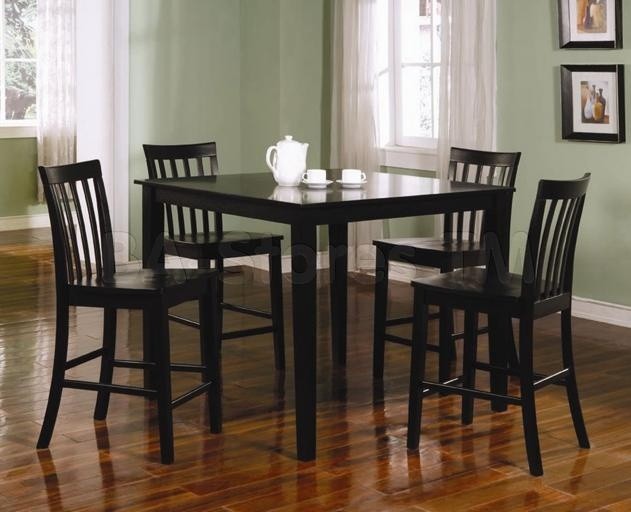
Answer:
[266,135,309,186]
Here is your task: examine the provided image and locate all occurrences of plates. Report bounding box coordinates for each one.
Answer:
[336,179,367,188]
[301,180,334,188]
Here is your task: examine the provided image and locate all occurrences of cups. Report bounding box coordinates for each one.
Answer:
[302,169,326,183]
[342,169,366,181]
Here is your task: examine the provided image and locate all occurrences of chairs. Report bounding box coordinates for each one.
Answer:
[35,160,223,462]
[144,142,286,410]
[370,146,521,404]
[406,173,592,476]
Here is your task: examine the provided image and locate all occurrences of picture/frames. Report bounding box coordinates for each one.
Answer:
[557,0,623,50]
[560,64,626,143]
